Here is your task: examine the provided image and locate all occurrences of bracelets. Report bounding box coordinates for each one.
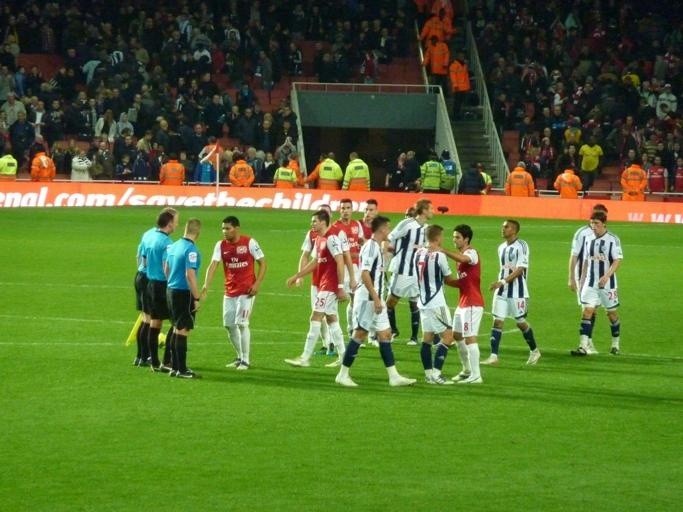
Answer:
[498,278,506,284]
[194,297,199,301]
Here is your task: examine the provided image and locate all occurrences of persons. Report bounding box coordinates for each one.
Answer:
[360,0,682,202]
[199,216,267,371]
[570,212,625,356]
[164,218,202,378]
[2,1,372,203]
[479,218,543,367]
[567,205,610,356]
[281,197,487,388]
[134,206,179,369]
[145,212,176,372]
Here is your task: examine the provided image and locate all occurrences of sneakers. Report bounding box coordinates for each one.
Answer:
[424,376,435,384]
[132,356,201,379]
[334,373,358,388]
[571,344,617,356]
[479,353,497,366]
[314,331,417,367]
[450,369,470,382]
[457,373,483,385]
[225,358,250,372]
[526,348,541,366]
[283,356,309,367]
[430,375,453,386]
[388,375,417,387]
